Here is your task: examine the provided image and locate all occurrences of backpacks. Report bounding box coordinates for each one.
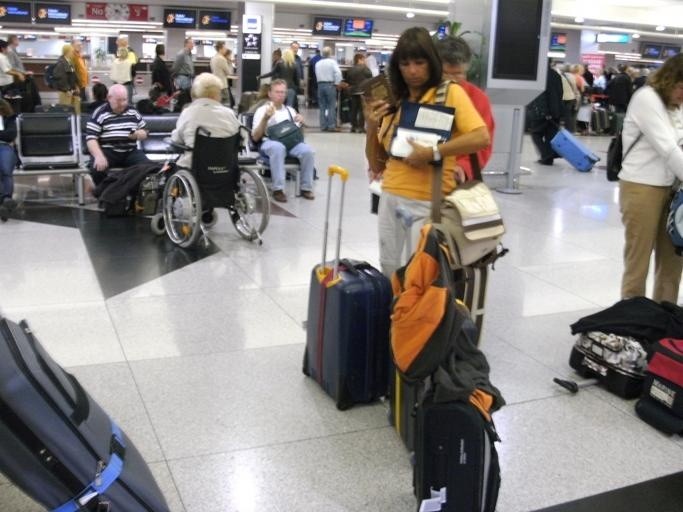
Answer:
[606,122,643,182]
[43,59,65,89]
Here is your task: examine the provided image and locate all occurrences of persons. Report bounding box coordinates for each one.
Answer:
[358,27,490,280]
[557,57,657,134]
[432,34,496,185]
[344,53,373,132]
[365,52,379,77]
[1,32,342,219]
[530,58,564,168]
[618,53,682,305]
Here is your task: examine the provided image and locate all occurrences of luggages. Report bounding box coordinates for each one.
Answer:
[452,260,488,339]
[302,162,396,413]
[383,295,480,454]
[549,124,602,173]
[596,107,610,130]
[238,89,262,114]
[408,375,502,512]
[608,112,625,134]
[0,315,172,512]
[553,327,648,401]
[590,110,601,132]
[633,336,683,437]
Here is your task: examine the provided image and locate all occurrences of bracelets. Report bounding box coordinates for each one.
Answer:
[431,143,442,165]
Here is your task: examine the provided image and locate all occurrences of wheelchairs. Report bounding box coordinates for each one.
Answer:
[161,127,268,248]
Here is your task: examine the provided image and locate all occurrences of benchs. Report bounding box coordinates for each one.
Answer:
[15,111,301,206]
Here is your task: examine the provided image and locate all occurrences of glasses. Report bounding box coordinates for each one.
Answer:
[440,73,467,83]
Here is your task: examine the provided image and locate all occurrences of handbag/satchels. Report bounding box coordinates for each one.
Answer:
[574,94,582,112]
[267,119,303,151]
[432,175,508,268]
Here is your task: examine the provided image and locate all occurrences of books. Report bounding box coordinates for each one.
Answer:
[357,72,397,116]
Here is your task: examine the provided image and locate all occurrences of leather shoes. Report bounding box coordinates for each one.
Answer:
[300,190,315,200]
[274,190,287,202]
